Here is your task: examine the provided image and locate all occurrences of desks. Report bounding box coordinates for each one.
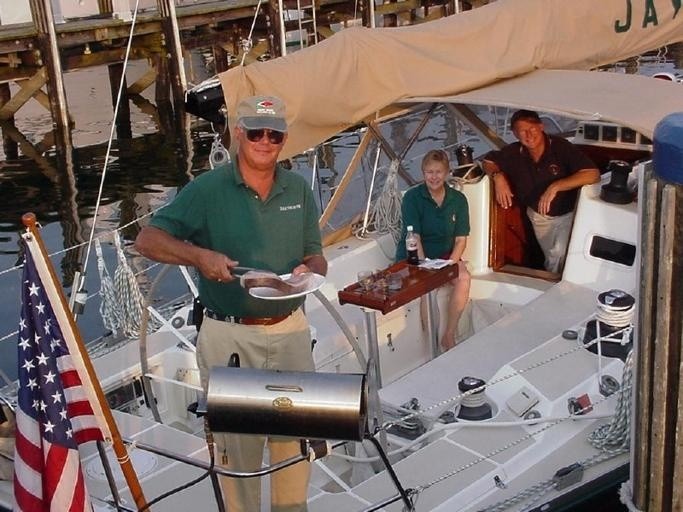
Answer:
[338,255,459,389]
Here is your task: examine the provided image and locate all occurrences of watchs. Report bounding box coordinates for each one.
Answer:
[491,169,502,177]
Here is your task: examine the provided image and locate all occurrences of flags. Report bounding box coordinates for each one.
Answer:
[13,250,105,512]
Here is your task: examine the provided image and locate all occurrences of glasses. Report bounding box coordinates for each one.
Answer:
[246,129,284,144]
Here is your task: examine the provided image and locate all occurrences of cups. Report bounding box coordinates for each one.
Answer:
[386,273,402,290]
[358,271,374,291]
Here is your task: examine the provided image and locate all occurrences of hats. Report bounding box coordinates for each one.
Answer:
[235,96,288,134]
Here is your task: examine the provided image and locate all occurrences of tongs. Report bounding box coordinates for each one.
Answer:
[231,261,264,283]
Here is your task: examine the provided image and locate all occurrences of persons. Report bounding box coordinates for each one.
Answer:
[395,150,470,350]
[482,110,601,274]
[134,97,327,511]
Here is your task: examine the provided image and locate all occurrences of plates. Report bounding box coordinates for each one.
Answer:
[249,271,326,300]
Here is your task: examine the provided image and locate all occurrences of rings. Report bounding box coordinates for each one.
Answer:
[218,279,221,281]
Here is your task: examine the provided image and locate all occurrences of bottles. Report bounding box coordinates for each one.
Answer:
[404,225,419,265]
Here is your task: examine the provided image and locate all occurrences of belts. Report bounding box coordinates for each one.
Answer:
[206,308,296,325]
